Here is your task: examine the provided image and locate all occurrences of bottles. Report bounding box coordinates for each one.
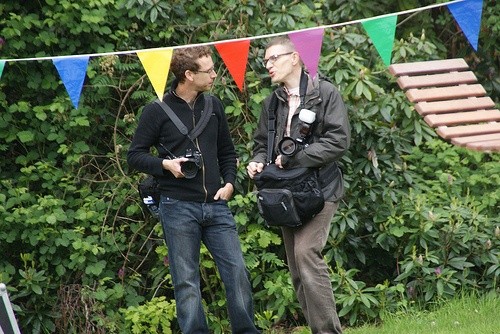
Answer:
[143,194,160,218]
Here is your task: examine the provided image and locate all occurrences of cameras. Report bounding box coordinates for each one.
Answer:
[277,109,316,155]
[178,148,202,179]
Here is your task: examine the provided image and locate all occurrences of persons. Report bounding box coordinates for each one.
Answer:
[127,46,259,334]
[247,37,351,334]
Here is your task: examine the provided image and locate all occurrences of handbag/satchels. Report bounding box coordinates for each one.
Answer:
[253,162,324,228]
[138,174,159,219]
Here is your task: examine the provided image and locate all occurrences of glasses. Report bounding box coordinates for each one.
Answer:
[194,67,216,74]
[262,52,295,68]
